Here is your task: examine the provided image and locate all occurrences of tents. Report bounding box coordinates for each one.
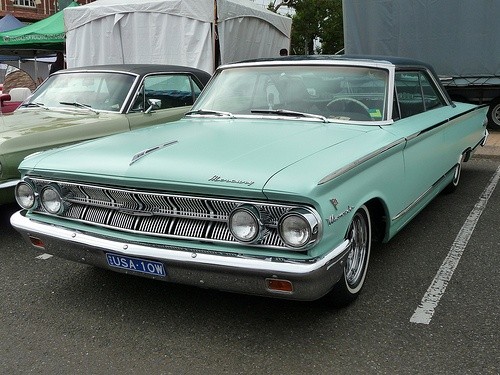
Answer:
[63,0,293,76]
[0,2,81,83]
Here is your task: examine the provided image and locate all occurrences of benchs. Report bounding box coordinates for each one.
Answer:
[132,89,199,111]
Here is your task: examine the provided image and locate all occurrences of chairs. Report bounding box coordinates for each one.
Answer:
[350,99,400,118]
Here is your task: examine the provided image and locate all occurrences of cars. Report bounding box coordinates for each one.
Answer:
[9,55,490,302]
[0,64,249,204]
